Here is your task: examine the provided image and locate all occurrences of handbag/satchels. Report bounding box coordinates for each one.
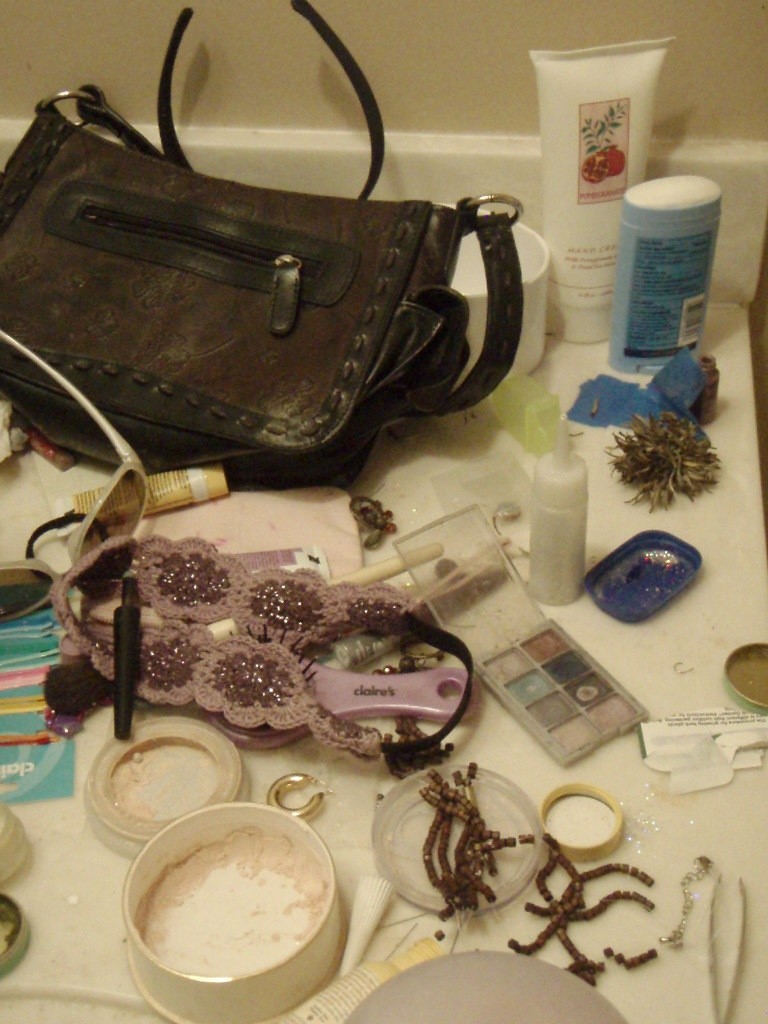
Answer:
[0,0,525,492]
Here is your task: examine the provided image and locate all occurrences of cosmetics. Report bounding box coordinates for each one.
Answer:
[392,506,648,767]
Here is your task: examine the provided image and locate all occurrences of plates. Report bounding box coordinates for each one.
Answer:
[345,951,627,1024]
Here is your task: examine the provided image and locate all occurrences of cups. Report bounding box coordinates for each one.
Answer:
[437,203,550,385]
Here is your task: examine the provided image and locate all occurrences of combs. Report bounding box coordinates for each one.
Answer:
[204,624,480,750]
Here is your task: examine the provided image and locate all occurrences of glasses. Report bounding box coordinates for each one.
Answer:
[0,330,149,622]
